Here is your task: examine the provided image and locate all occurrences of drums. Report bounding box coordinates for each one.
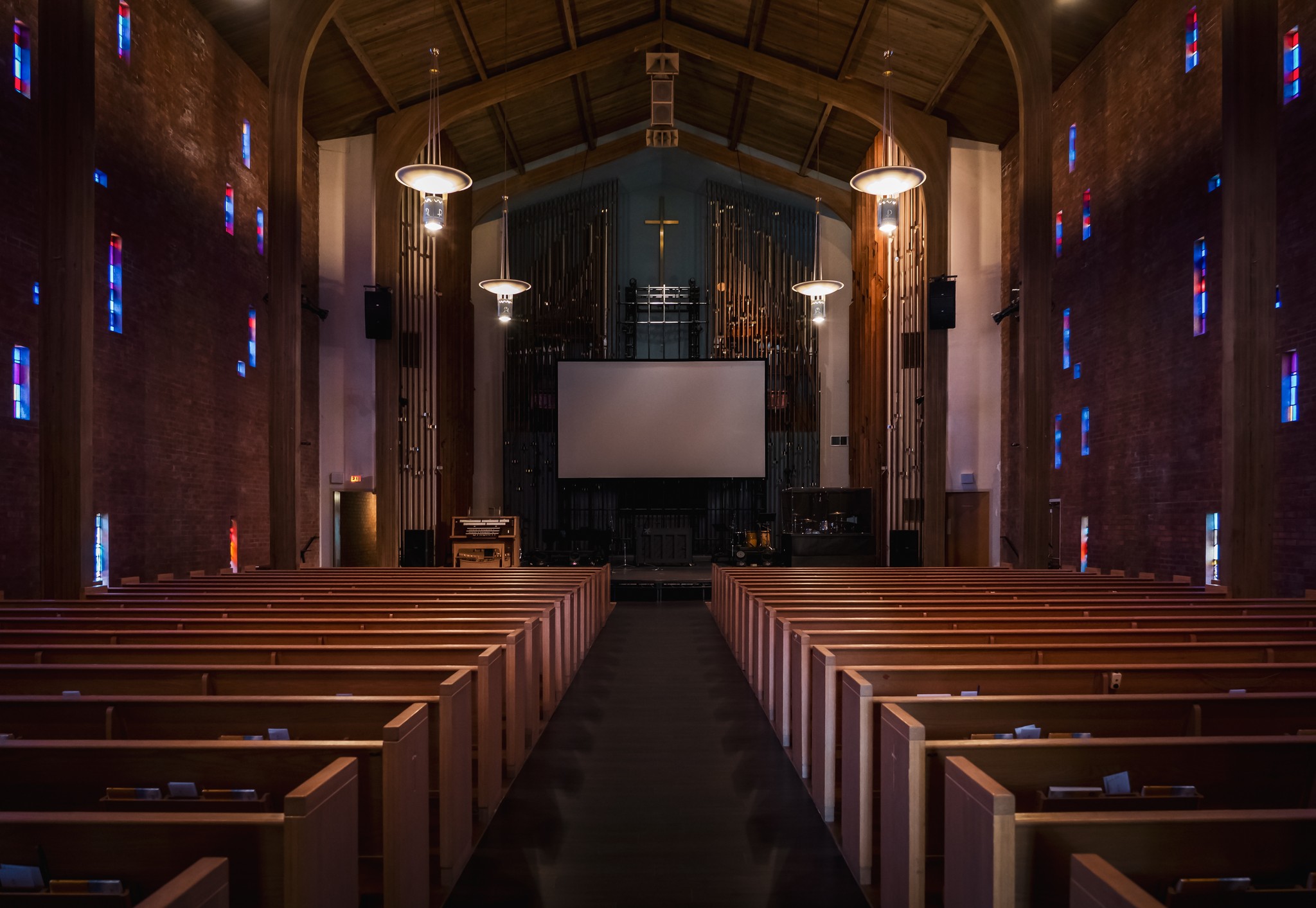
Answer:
[819,520,828,530]
[804,529,822,534]
[734,532,745,547]
[746,531,758,548]
[760,531,771,548]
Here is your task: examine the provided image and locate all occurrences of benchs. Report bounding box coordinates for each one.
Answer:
[0,569,612,908]
[706,563,1316,908]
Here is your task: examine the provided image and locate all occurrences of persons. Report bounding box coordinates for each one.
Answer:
[1048,553,1060,569]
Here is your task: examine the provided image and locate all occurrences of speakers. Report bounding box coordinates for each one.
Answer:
[651,75,674,126]
[890,530,921,568]
[403,529,435,568]
[363,289,393,341]
[929,280,956,329]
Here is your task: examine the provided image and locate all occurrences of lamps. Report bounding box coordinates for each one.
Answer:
[395,48,473,233]
[479,0,531,322]
[792,0,845,323]
[850,0,926,231]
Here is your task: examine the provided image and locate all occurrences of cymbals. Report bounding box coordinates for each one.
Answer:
[828,512,846,515]
[798,519,818,523]
[790,513,802,516]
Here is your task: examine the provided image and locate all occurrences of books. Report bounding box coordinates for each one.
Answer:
[0,734,16,740]
[1306,873,1316,891]
[1176,878,1250,893]
[221,735,263,741]
[106,783,257,800]
[48,880,122,892]
[971,733,1089,740]
[1048,772,1195,798]
